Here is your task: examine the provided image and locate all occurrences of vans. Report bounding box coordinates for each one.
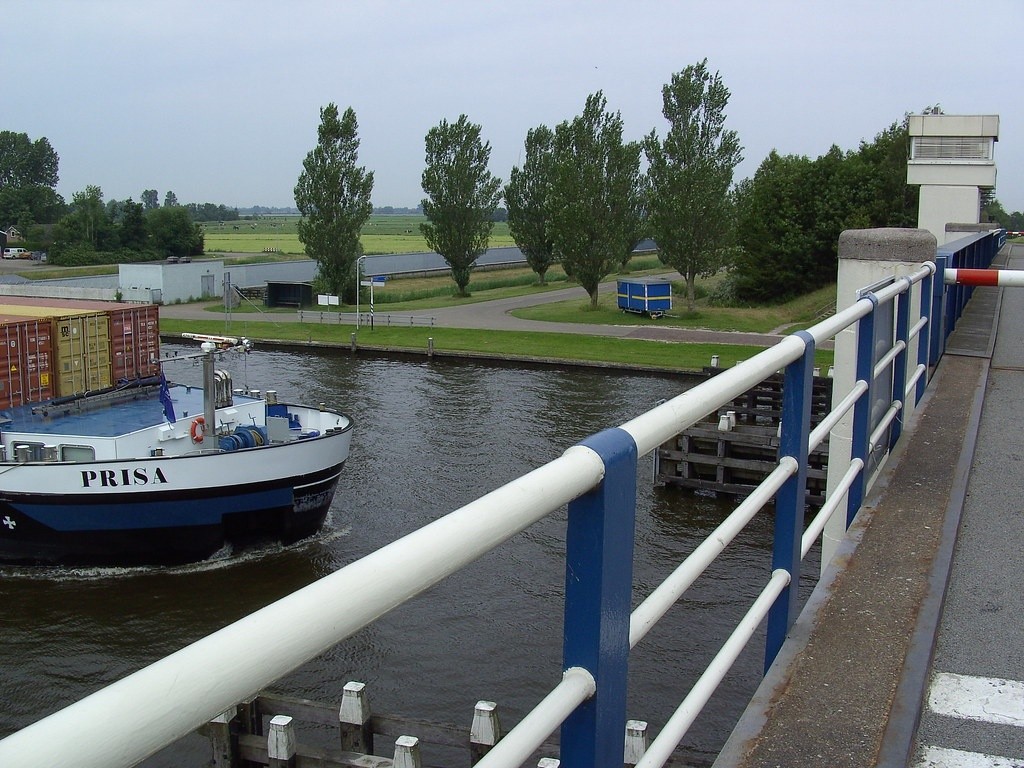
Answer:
[3,248,28,259]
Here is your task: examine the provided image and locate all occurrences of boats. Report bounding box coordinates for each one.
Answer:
[0,292,355,567]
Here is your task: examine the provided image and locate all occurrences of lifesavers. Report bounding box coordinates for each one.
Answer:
[190,417,204,442]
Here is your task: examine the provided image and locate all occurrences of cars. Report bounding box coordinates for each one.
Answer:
[19,251,48,261]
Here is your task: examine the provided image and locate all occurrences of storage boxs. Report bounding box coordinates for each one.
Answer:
[0,292,163,418]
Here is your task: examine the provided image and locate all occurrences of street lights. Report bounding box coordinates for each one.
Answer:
[355,253,368,330]
